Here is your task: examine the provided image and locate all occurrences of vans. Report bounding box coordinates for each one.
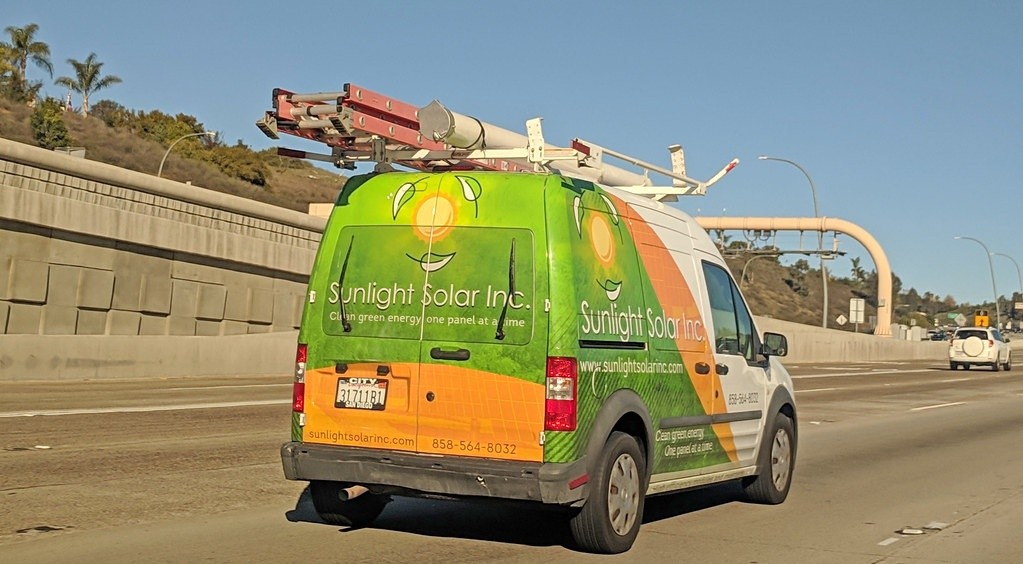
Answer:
[277,171,800,557]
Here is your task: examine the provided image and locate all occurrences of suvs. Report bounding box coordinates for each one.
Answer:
[948,327,1012,372]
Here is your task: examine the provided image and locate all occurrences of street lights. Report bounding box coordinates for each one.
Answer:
[759,157,829,328]
[953,236,1001,326]
[989,252,1022,294]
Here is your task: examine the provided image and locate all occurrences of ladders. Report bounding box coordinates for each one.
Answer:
[271,81,533,173]
